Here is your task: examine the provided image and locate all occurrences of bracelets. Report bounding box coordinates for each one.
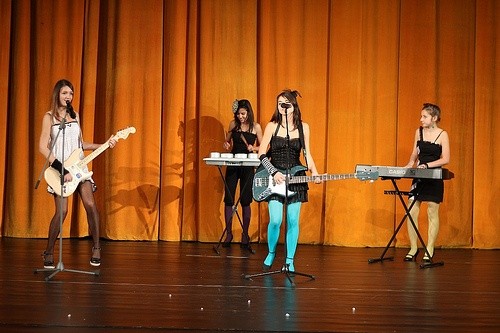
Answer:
[424,163,429,168]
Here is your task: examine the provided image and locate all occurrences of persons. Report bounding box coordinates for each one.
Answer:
[39,79,119,267]
[403,103,451,260]
[222,99,264,247]
[259,89,327,275]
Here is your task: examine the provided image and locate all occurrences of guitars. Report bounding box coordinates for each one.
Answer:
[252,165,380,202]
[44,125,136,197]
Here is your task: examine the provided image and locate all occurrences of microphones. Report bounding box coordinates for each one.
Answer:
[66,100,76,119]
[281,102,292,108]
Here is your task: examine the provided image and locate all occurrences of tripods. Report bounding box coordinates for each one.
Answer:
[244,108,315,287]
[32,110,100,281]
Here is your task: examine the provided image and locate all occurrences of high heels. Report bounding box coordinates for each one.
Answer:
[422,248,435,262]
[404,248,420,261]
[285,257,295,277]
[42,250,56,268]
[90,245,101,266]
[222,232,233,247]
[263,251,277,269]
[241,233,250,248]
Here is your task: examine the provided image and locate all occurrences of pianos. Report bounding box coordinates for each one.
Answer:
[355,164,455,268]
